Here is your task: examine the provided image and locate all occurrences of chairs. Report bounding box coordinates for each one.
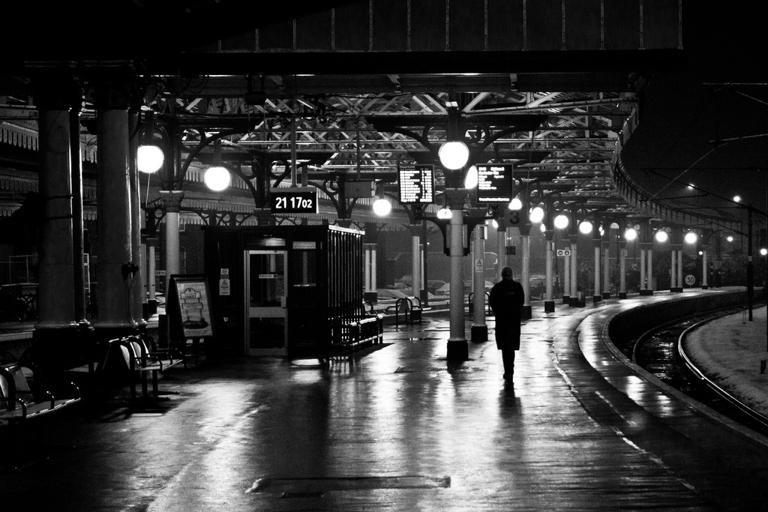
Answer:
[68,333,183,414]
[0,362,82,471]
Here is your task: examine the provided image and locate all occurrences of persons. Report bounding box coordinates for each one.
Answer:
[487,267,525,383]
